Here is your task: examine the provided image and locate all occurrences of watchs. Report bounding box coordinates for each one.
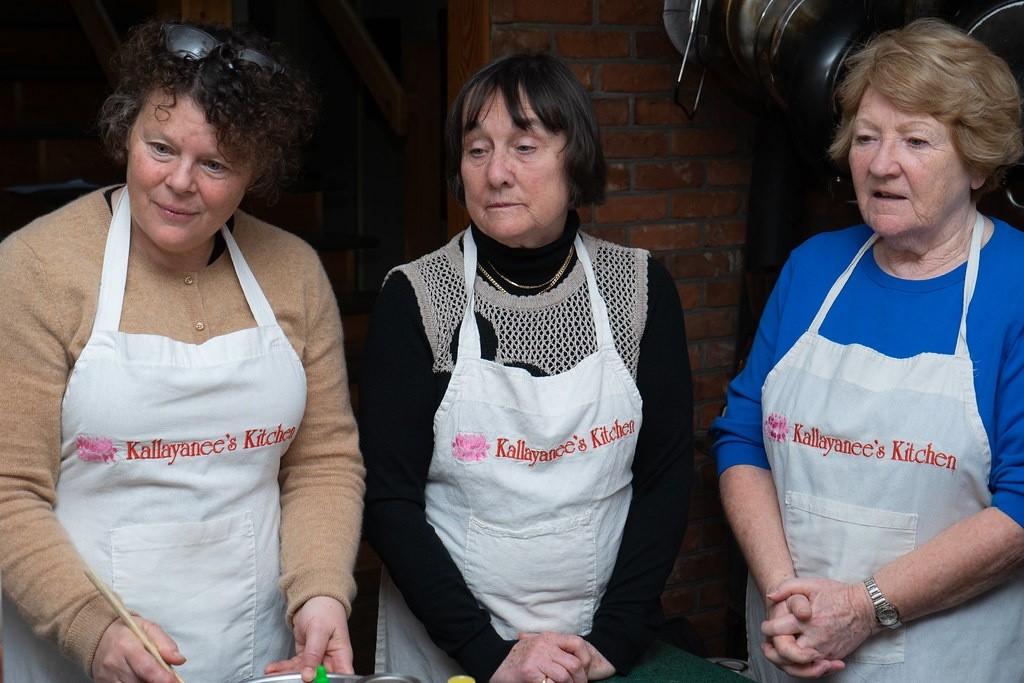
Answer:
[863,576,904,630]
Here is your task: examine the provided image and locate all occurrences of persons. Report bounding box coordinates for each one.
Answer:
[358,49,761,681]
[705,17,1024,683]
[0,20,370,683]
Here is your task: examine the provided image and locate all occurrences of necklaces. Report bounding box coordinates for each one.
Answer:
[460,229,575,297]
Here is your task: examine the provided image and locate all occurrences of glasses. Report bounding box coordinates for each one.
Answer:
[158,23,288,87]
